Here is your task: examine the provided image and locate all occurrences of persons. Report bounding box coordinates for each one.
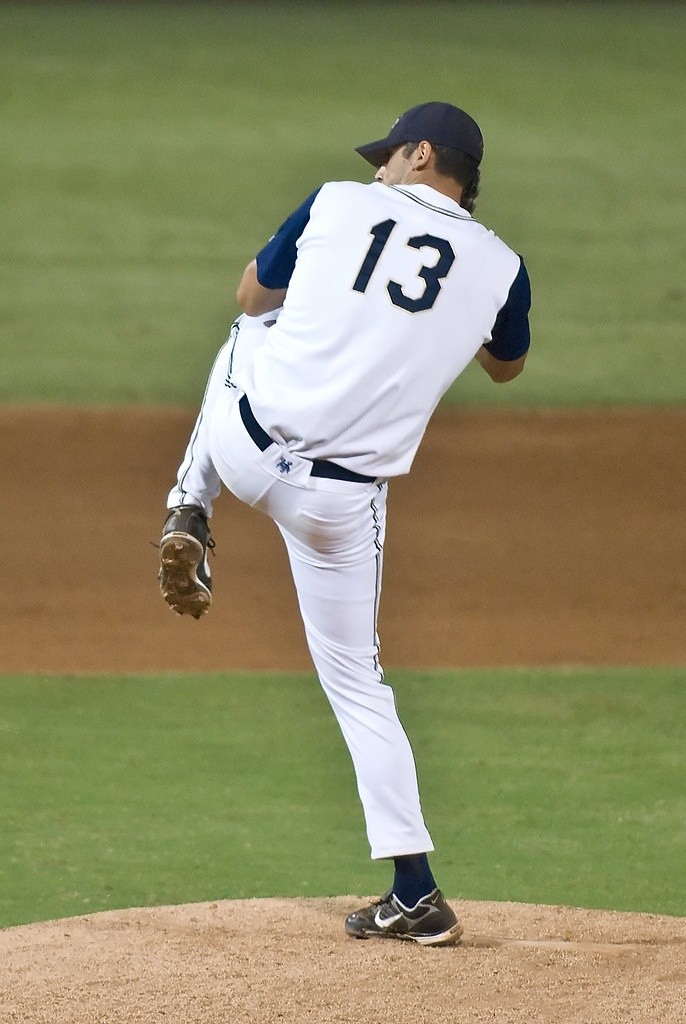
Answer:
[159,101,530,947]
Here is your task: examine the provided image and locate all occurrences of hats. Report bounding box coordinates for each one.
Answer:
[355,102,484,169]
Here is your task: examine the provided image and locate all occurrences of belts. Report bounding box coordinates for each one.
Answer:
[238,393,377,484]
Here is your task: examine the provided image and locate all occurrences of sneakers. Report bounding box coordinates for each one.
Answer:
[150,508,213,619]
[345,885,462,946]
